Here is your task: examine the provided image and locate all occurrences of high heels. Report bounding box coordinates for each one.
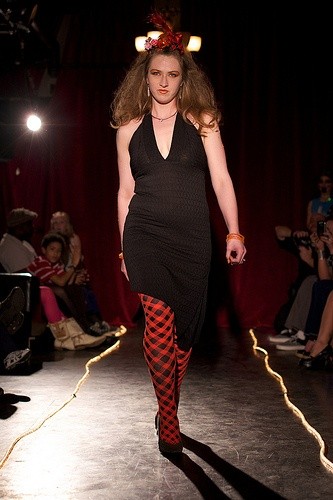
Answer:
[299,344,331,367]
[155,412,183,458]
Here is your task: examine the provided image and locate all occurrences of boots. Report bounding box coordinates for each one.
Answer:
[50,319,76,351]
[64,318,106,350]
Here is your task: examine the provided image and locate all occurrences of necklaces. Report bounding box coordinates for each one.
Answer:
[151,109,179,122]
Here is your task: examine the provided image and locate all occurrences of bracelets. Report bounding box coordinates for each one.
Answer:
[225,233,245,243]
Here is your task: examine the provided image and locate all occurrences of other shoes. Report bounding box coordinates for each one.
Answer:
[297,349,311,358]
[90,320,110,334]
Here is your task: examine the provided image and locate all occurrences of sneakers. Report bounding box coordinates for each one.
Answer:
[276,337,307,350]
[268,333,292,343]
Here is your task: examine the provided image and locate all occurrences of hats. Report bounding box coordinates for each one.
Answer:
[8,208,38,227]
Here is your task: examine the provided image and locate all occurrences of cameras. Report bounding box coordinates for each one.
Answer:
[316,222,326,237]
[296,236,311,248]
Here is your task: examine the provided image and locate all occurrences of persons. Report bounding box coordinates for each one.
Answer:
[109,11,248,458]
[265,165,333,359]
[0,207,121,349]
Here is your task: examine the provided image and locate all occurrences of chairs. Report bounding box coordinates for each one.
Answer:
[0,270,41,374]
